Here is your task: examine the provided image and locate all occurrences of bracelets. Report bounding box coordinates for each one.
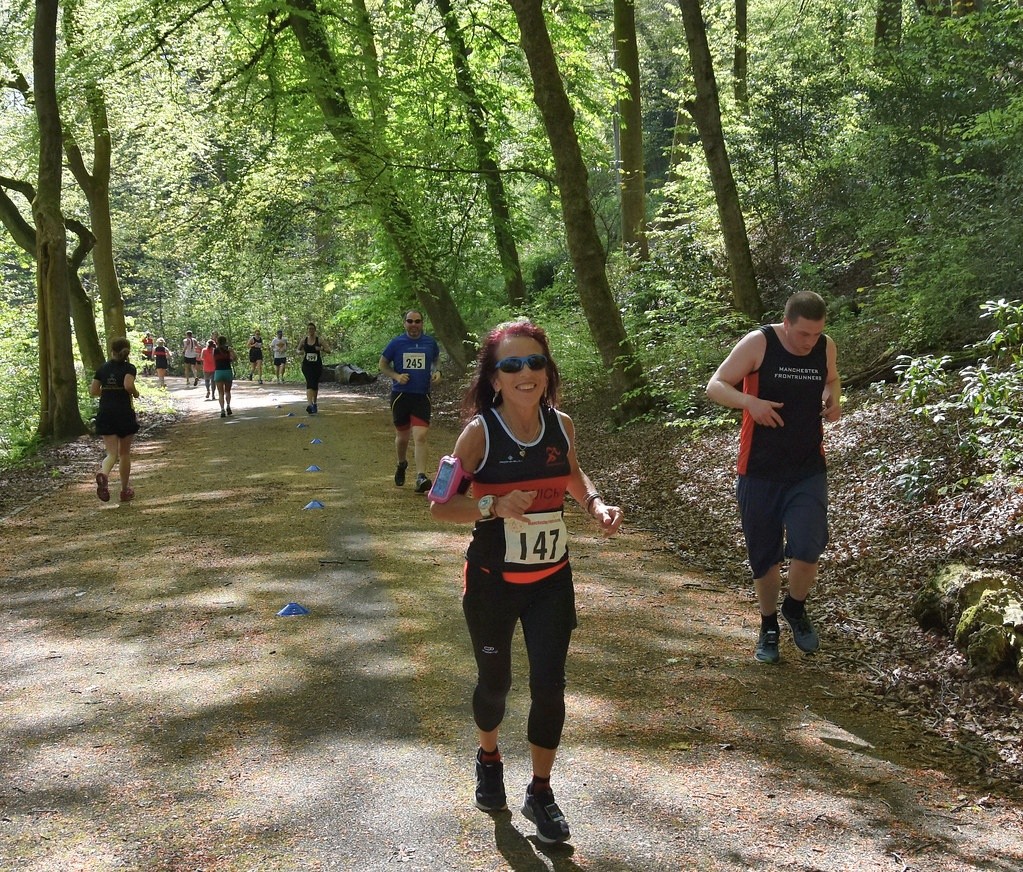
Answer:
[585,493,603,515]
[435,369,442,376]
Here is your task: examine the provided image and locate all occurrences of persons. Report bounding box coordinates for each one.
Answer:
[298,323,331,414]
[271,330,288,384]
[213,336,234,418]
[201,339,216,399]
[427,321,624,842]
[90,338,139,502]
[182,330,198,386]
[143,333,153,376]
[196,343,202,370]
[211,331,218,342]
[706,291,841,662]
[248,330,263,384]
[379,310,442,493]
[152,337,172,388]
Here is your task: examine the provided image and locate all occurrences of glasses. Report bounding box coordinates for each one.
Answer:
[404,318,422,324]
[494,353,548,373]
[307,328,315,331]
[255,333,260,335]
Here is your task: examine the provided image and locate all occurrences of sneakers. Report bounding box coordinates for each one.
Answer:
[96,473,110,502]
[415,474,433,492]
[754,623,784,663]
[121,488,135,501]
[474,748,509,811]
[520,783,571,843]
[394,461,408,486]
[781,601,819,652]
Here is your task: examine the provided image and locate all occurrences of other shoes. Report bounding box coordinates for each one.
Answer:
[206,391,210,398]
[259,380,263,384]
[220,412,226,417]
[212,395,215,399]
[194,377,198,386]
[187,381,190,385]
[226,408,232,415]
[306,403,317,414]
[250,374,252,381]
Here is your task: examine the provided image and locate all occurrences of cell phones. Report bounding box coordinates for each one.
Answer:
[430,460,459,499]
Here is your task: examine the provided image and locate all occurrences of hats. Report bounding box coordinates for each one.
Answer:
[278,330,282,335]
